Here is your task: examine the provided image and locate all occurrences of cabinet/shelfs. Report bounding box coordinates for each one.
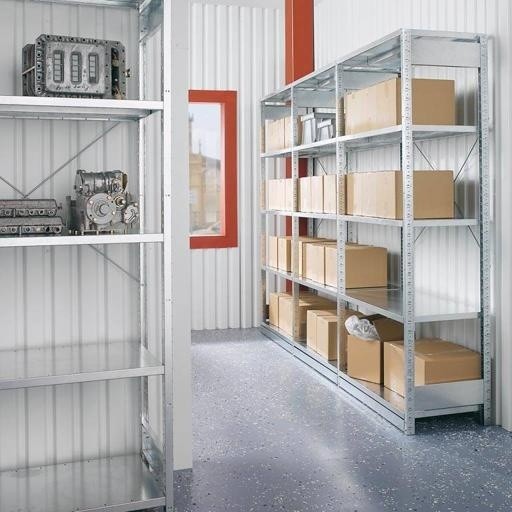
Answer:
[257,28,496,436]
[0,0,175,512]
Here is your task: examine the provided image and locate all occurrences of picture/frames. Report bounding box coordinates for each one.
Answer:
[188,89,237,248]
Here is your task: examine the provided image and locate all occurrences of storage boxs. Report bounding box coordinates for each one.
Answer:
[269,290,366,361]
[263,174,337,214]
[261,235,388,289]
[343,78,456,136]
[346,169,454,220]
[265,117,301,153]
[346,318,404,384]
[383,337,482,399]
